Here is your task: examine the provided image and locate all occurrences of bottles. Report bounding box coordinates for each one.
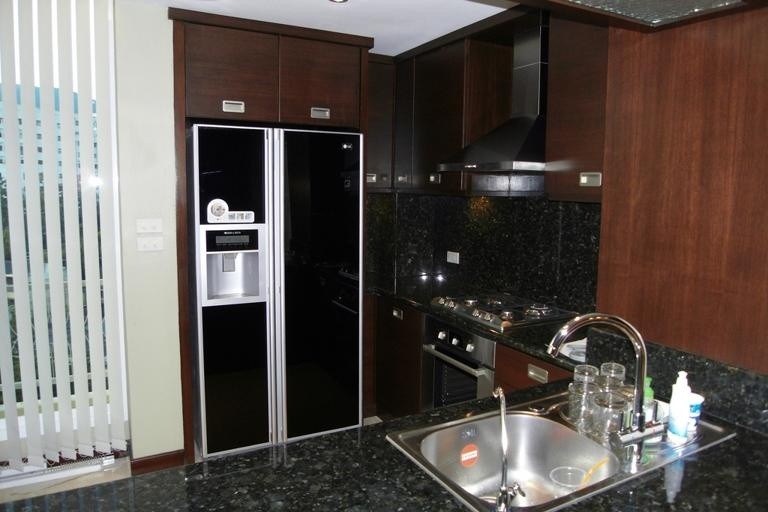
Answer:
[666,370,691,444]
[664,443,686,504]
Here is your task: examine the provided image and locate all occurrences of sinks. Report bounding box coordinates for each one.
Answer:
[383,408,620,511]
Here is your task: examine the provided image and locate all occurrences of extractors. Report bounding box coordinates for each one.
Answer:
[437,14,548,175]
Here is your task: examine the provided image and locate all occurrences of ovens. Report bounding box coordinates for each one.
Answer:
[418,313,497,413]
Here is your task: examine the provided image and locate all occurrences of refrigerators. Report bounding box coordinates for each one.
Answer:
[185,122,364,461]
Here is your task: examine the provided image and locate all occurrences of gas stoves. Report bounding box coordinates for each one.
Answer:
[430,290,581,334]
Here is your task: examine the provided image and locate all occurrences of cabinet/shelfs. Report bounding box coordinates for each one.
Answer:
[494,341,574,392]
[167,8,375,130]
[374,300,434,415]
[369,0,608,204]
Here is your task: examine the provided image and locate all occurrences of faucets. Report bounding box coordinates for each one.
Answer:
[492,386,526,511]
[545,312,664,461]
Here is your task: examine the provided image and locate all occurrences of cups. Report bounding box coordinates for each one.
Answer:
[548,466,591,499]
[688,393,706,431]
[567,362,635,442]
